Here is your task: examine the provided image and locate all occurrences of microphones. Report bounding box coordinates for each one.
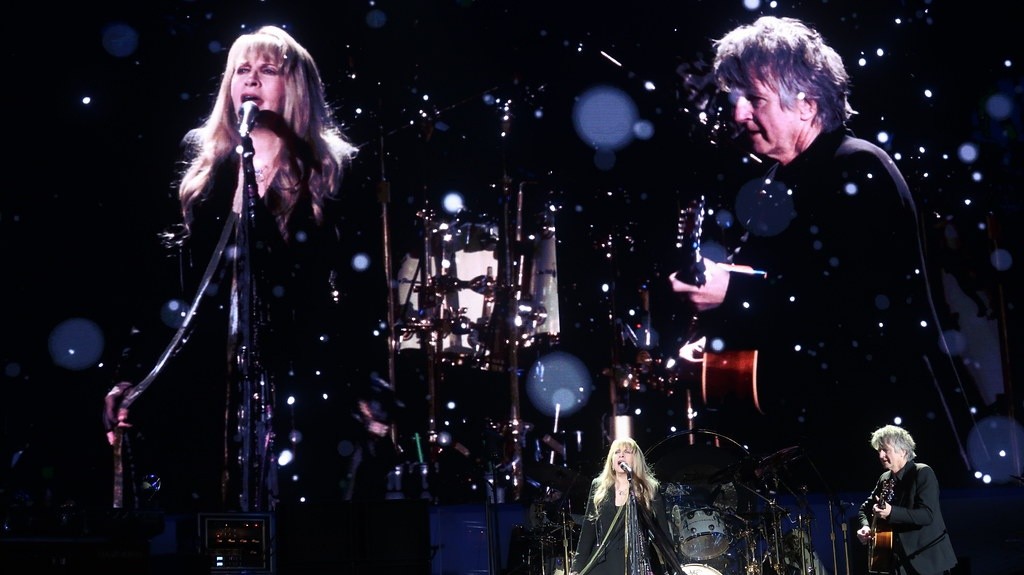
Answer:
[620,462,631,472]
[236,101,260,140]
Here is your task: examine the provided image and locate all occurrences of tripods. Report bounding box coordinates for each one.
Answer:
[341,83,575,505]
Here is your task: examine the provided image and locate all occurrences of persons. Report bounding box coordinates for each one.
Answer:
[565,437,667,575]
[857,425,960,575]
[104,25,432,575]
[666,15,976,479]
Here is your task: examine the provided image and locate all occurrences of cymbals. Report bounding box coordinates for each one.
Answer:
[750,445,801,482]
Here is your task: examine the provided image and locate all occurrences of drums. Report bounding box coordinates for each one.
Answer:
[667,561,738,575]
[676,507,732,560]
[385,215,565,353]
[542,553,576,575]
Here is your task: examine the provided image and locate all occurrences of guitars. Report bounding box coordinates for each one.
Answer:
[673,194,771,418]
[867,479,896,575]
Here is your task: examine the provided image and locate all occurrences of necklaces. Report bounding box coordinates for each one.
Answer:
[616,488,628,496]
[249,161,275,184]
